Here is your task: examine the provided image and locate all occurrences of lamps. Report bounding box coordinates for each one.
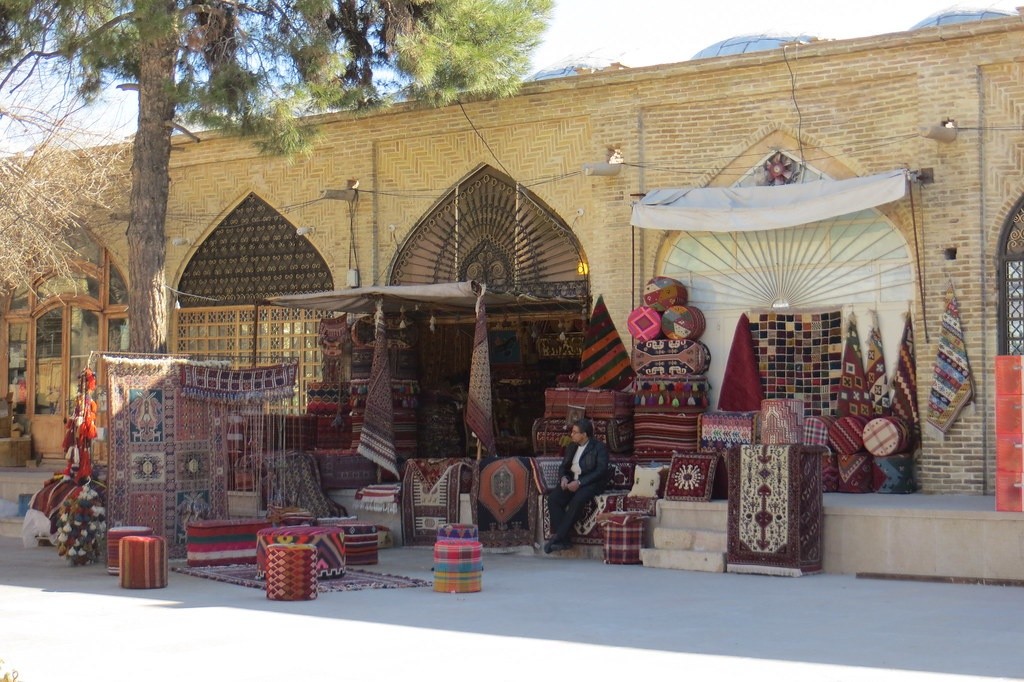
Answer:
[296,226,317,247]
[171,238,207,250]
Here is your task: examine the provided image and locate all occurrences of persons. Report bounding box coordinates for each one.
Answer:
[544,419,609,553]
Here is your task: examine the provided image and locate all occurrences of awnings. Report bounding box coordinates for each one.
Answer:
[630,168,931,367]
[263,281,515,307]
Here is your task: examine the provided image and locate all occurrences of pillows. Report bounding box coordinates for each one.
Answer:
[625,463,668,499]
[662,453,719,504]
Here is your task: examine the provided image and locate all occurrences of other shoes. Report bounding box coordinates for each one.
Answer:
[544,535,560,554]
[551,538,572,551]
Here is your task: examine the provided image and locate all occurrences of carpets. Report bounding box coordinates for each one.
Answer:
[170,567,435,595]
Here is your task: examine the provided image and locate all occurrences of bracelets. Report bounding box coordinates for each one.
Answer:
[578,480,581,485]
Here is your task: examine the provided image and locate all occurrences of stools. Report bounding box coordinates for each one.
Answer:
[837,454,872,493]
[600,515,649,564]
[255,526,344,581]
[437,523,478,541]
[661,306,706,339]
[822,453,838,493]
[435,541,482,592]
[336,522,379,565]
[106,526,153,576]
[644,276,687,313]
[860,415,914,456]
[628,306,666,342]
[761,399,803,445]
[119,535,168,588]
[803,416,837,447]
[185,519,273,566]
[871,454,915,495]
[265,542,318,601]
[830,415,872,454]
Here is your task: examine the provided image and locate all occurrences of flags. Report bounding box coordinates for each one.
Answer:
[717,311,765,413]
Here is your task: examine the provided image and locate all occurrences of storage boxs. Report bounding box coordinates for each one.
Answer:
[0,438,32,466]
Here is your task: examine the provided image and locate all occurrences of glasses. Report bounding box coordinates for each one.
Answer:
[571,429,581,434]
